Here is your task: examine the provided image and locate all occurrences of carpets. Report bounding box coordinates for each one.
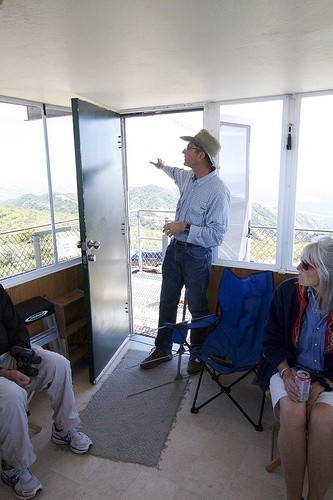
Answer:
[61,347,192,468]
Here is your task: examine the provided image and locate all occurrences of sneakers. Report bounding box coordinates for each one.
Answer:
[140,348,172,370]
[1,460,42,500]
[51,422,93,454]
[187,356,205,374]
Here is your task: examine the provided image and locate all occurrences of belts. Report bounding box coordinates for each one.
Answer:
[174,238,202,247]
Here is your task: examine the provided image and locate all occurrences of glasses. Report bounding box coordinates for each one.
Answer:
[186,144,202,152]
[301,260,316,271]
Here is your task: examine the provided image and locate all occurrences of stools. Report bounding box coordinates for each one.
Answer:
[14,289,87,356]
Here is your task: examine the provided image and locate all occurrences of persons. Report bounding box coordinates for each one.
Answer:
[261,236,333,500]
[0,284,93,500]
[140,129,230,374]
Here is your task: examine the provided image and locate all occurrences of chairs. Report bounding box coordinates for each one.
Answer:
[164,272,284,471]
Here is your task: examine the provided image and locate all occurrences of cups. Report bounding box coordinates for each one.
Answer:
[165,219,173,235]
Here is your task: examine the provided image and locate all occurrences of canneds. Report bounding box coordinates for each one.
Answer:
[294,370,311,402]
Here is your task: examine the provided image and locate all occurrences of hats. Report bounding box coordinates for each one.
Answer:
[179,128,222,170]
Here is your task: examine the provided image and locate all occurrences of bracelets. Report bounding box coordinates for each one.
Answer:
[317,378,332,392]
[280,368,288,378]
[184,224,191,235]
